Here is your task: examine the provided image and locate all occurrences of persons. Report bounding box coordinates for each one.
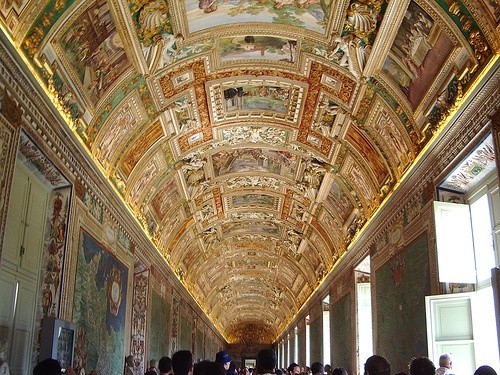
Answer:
[32,359,61,375]
[124,349,457,375]
[474,365,497,375]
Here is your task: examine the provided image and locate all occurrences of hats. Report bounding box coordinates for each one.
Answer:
[216,351,232,363]
